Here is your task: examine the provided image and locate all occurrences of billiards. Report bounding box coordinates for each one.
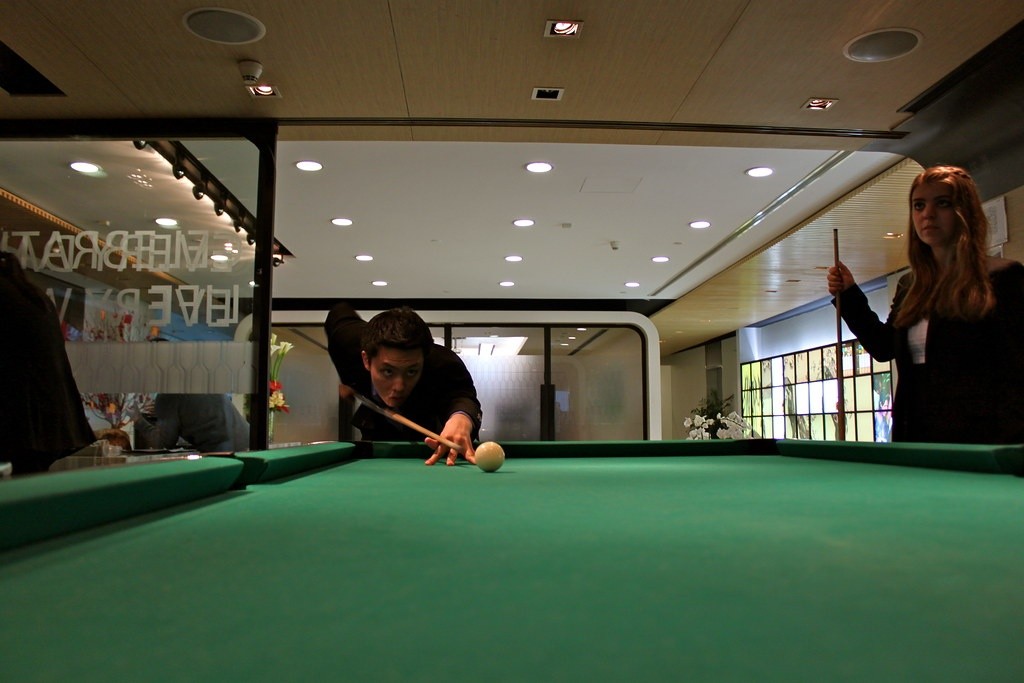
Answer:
[472,442,505,474]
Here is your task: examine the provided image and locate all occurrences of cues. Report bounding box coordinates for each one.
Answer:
[830,226,848,441]
[340,389,464,453]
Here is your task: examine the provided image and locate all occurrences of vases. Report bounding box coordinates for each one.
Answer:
[267,411,273,445]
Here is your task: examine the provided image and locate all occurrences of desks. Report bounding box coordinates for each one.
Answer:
[0,438,1024,683]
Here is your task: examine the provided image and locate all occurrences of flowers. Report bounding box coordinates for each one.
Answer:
[243,333,294,444]
[684,388,755,440]
[64,310,161,429]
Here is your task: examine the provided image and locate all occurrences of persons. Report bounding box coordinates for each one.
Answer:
[0,250,98,479]
[325,305,482,467]
[126,337,249,453]
[826,167,1024,447]
